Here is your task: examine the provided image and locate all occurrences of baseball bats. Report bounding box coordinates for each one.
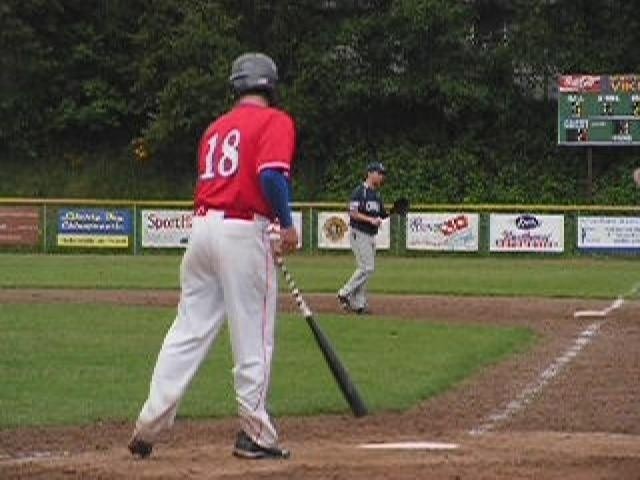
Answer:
[277,254,369,418]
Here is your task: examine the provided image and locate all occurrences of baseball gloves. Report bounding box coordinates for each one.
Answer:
[393,197,411,216]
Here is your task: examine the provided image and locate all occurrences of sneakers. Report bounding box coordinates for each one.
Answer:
[335,291,373,315]
[231,429,290,460]
[126,432,152,458]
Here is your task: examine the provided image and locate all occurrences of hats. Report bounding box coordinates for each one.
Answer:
[365,162,388,177]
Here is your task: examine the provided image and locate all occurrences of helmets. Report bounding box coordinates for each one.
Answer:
[228,52,280,95]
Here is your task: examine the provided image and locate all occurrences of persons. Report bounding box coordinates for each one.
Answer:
[337,160,390,315]
[129,52,298,460]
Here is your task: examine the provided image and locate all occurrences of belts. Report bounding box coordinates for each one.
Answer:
[195,207,274,222]
